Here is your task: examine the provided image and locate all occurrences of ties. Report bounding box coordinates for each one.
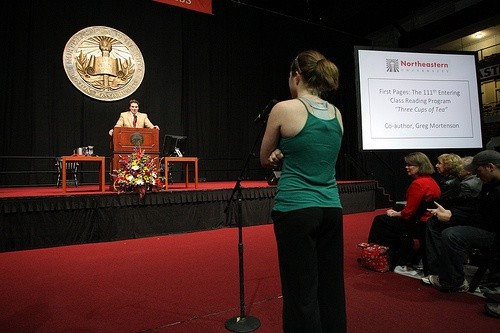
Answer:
[133,114,137,128]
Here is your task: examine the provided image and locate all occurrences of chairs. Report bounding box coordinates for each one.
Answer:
[54,157,79,188]
[159,152,173,184]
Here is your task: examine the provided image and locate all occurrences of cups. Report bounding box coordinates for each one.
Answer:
[89,146,95,154]
[78,148,82,155]
[83,147,89,155]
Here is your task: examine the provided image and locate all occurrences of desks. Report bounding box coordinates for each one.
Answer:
[158,156,198,191]
[61,156,106,193]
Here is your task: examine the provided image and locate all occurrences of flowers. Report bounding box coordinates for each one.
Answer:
[110,145,167,198]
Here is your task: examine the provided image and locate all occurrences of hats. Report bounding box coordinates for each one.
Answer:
[468,150,500,169]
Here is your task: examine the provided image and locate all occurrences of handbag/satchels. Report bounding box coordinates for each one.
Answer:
[357,243,389,273]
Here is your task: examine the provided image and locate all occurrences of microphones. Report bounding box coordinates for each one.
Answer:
[254,98,278,123]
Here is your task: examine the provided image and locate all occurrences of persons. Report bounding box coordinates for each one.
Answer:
[260,51,348,333]
[109,100,160,136]
[367,150,500,292]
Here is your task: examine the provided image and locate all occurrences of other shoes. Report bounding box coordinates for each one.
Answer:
[486,303,500,318]
[421,274,469,292]
